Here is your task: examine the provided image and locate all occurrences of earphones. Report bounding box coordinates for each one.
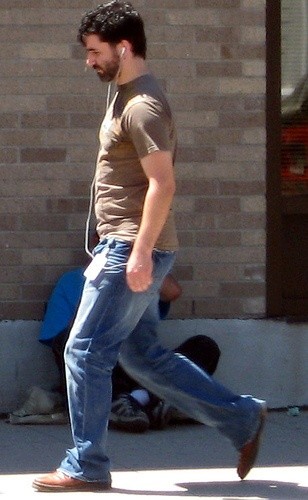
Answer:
[122,47,126,54]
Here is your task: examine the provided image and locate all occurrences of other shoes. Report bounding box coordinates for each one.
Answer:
[107,395,148,431]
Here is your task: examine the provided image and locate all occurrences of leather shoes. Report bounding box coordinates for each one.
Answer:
[237,398,268,480]
[34,470,111,492]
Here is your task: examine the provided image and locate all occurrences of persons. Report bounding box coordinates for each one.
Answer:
[32,0,267,494]
[36,225,221,434]
